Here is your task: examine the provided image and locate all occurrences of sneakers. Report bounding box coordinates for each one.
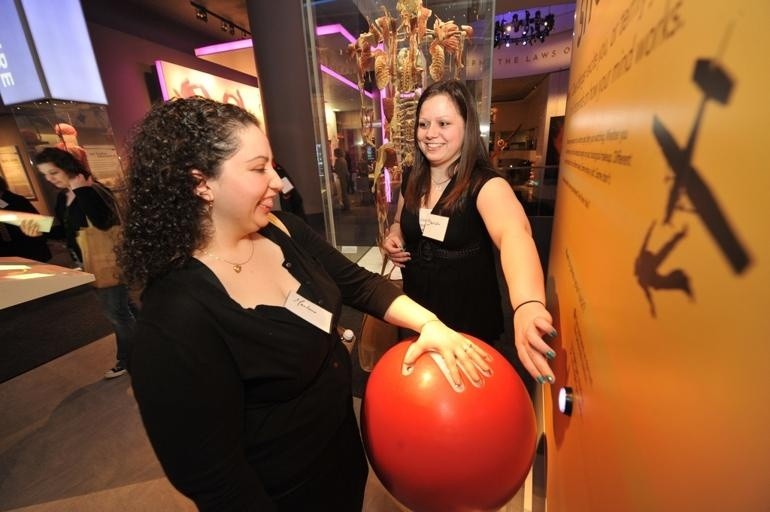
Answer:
[103,366,127,378]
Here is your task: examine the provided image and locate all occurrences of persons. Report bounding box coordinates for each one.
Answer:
[114,94,492,512]
[53,121,91,174]
[330,147,351,210]
[0,176,53,262]
[273,157,308,222]
[381,79,559,385]
[18,146,136,378]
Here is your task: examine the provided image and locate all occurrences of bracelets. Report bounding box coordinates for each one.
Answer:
[419,318,440,332]
[511,300,549,314]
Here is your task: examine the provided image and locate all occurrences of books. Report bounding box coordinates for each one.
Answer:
[1,204,54,236]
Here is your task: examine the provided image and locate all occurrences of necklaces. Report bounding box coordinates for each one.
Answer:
[194,237,255,275]
[430,175,454,188]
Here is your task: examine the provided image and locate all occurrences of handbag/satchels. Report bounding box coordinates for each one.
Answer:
[358,274,404,373]
[76,225,129,288]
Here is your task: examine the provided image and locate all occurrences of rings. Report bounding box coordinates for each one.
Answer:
[463,343,473,351]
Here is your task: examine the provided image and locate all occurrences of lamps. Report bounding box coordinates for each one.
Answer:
[190,2,251,40]
[494,4,555,49]
